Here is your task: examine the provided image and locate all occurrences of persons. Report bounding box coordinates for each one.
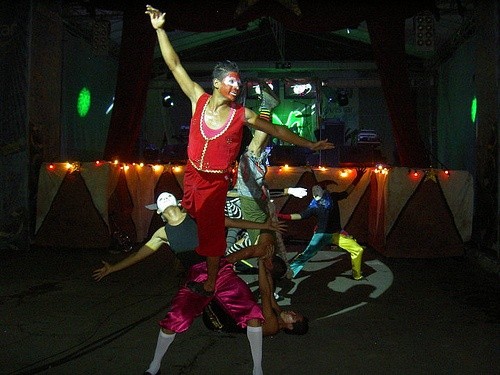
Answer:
[145,4,335,297]
[90,80,365,375]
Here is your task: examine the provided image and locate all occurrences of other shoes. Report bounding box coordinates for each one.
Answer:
[142,368,161,375]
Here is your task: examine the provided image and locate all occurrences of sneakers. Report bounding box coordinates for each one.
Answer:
[187,281,214,297]
[256,77,282,111]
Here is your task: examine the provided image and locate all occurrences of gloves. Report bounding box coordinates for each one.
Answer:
[276,209,291,221]
[353,165,367,186]
